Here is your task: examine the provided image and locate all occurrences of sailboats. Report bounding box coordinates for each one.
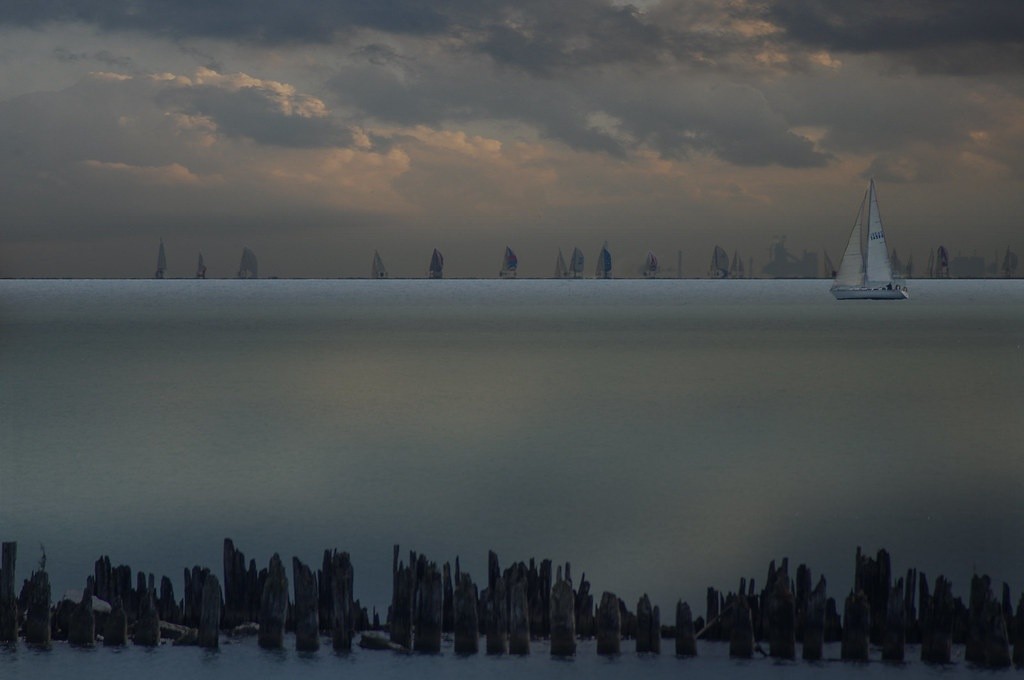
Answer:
[926,243,952,279]
[1000,244,1017,279]
[369,237,746,280]
[150,236,278,281]
[826,174,910,302]
